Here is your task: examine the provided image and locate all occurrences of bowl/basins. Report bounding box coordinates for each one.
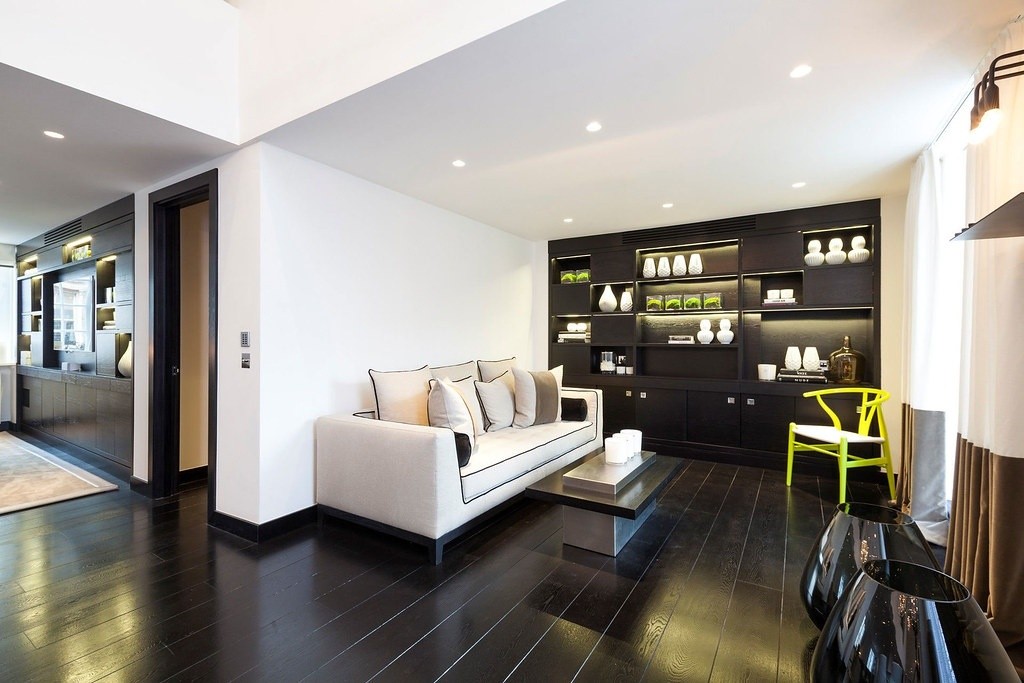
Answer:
[646,295,664,312]
[704,292,723,309]
[576,269,591,283]
[560,271,575,284]
[577,322,587,331]
[665,294,683,311]
[567,323,577,332]
[683,294,702,310]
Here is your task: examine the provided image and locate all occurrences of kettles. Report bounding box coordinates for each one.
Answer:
[600,350,618,375]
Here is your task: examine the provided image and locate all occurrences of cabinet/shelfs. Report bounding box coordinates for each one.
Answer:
[547,198,882,485]
[17,212,135,486]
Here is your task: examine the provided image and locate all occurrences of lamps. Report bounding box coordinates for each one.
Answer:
[967,49,1024,145]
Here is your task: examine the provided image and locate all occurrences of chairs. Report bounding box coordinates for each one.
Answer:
[785,387,897,504]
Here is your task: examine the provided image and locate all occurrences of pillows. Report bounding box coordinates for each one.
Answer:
[429,375,487,437]
[511,364,564,429]
[477,357,519,395]
[474,370,517,433]
[430,360,481,386]
[426,378,476,453]
[368,365,434,426]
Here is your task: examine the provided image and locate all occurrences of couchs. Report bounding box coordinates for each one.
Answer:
[315,358,607,567]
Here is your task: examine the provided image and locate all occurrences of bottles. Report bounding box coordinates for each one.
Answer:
[825,238,847,265]
[809,559,1022,683]
[697,319,714,344]
[688,254,703,275]
[672,255,687,276]
[598,284,617,312]
[118,341,131,379]
[799,503,943,633]
[620,291,633,312]
[643,257,656,278]
[717,319,734,344]
[803,346,820,371]
[804,239,824,267]
[657,257,671,277]
[848,236,870,264]
[784,346,801,370]
[829,335,866,384]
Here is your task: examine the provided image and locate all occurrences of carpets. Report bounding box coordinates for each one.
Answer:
[0,429,119,516]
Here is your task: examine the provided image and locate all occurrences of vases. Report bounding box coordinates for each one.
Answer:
[688,253,704,276]
[620,291,633,312]
[118,340,132,378]
[657,256,672,277]
[804,346,820,372]
[592,281,618,313]
[786,344,803,371]
[673,254,688,277]
[642,257,655,279]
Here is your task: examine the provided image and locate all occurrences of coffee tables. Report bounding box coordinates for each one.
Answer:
[525,445,686,558]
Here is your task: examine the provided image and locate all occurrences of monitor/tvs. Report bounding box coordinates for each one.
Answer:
[53,276,95,352]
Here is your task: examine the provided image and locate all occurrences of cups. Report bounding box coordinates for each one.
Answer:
[767,289,781,299]
[781,289,794,299]
[626,367,633,374]
[616,366,625,375]
[620,428,642,456]
[605,437,627,465]
[758,363,777,381]
[612,433,634,458]
